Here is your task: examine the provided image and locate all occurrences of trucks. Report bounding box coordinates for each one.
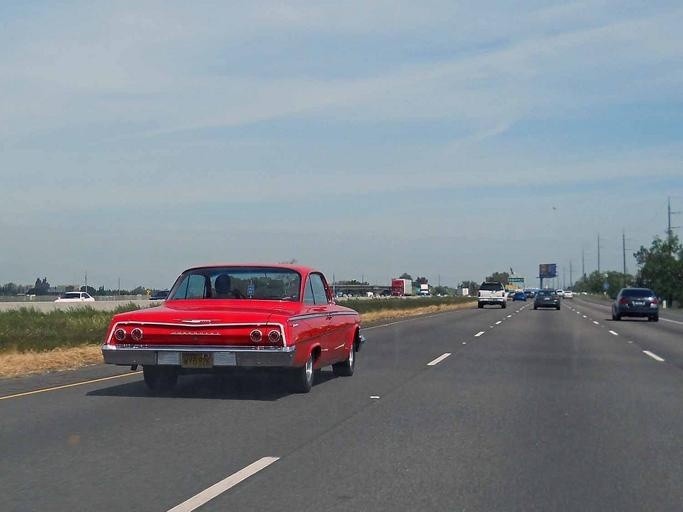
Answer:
[388,279,413,297]
[419,284,429,297]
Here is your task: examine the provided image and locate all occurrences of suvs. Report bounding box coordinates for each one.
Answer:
[477,281,506,309]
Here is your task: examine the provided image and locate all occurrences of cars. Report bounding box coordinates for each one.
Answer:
[335,291,344,297]
[532,289,559,310]
[553,288,587,298]
[608,286,661,323]
[51,290,94,304]
[506,288,535,301]
[149,289,170,299]
[96,263,364,395]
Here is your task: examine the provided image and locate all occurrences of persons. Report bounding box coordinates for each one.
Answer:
[212,275,232,299]
